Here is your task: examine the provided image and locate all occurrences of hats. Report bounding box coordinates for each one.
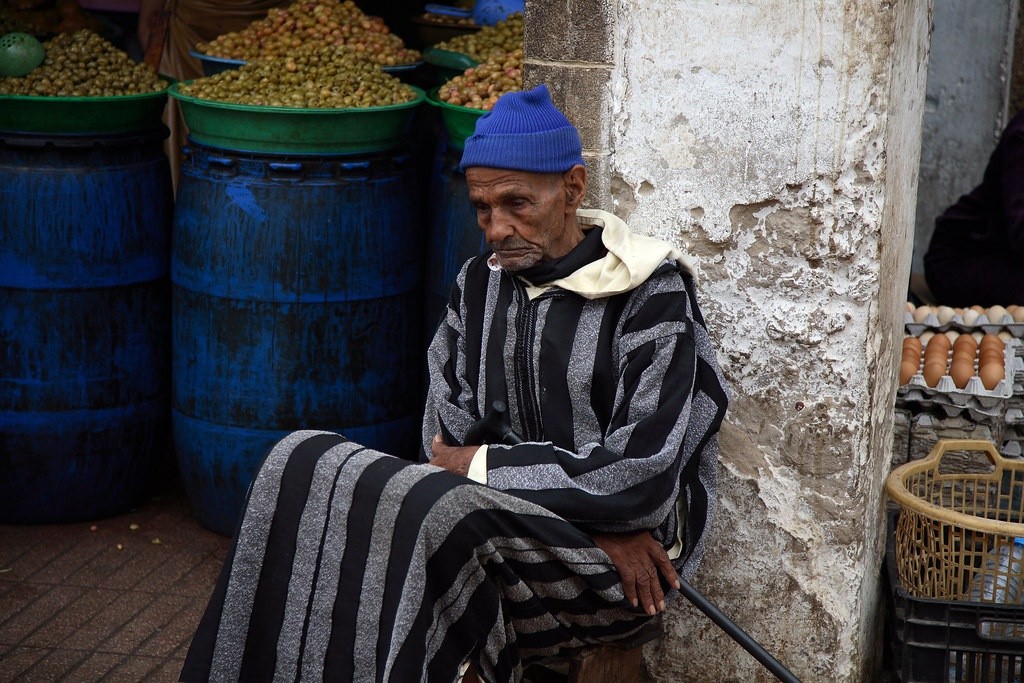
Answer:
[458,85,585,173]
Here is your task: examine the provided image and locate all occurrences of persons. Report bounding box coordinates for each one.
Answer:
[177,85,732,683]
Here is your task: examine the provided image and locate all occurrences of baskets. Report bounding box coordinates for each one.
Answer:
[887,438,1024,683]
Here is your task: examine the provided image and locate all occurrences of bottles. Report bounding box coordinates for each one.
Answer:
[945,535,1024,683]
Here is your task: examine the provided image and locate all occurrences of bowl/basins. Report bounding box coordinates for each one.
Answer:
[0,73,175,132]
[186,48,425,82]
[167,78,427,153]
[423,83,491,146]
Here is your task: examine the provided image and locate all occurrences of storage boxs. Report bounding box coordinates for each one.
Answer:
[884,509,1024,683]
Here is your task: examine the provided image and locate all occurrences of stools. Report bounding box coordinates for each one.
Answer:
[522,644,643,683]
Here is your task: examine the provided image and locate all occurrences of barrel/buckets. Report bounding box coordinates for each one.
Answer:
[1,123,174,524]
[410,144,492,323]
[172,137,434,538]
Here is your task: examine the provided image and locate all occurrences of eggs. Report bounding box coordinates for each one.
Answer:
[899,302,1024,391]
